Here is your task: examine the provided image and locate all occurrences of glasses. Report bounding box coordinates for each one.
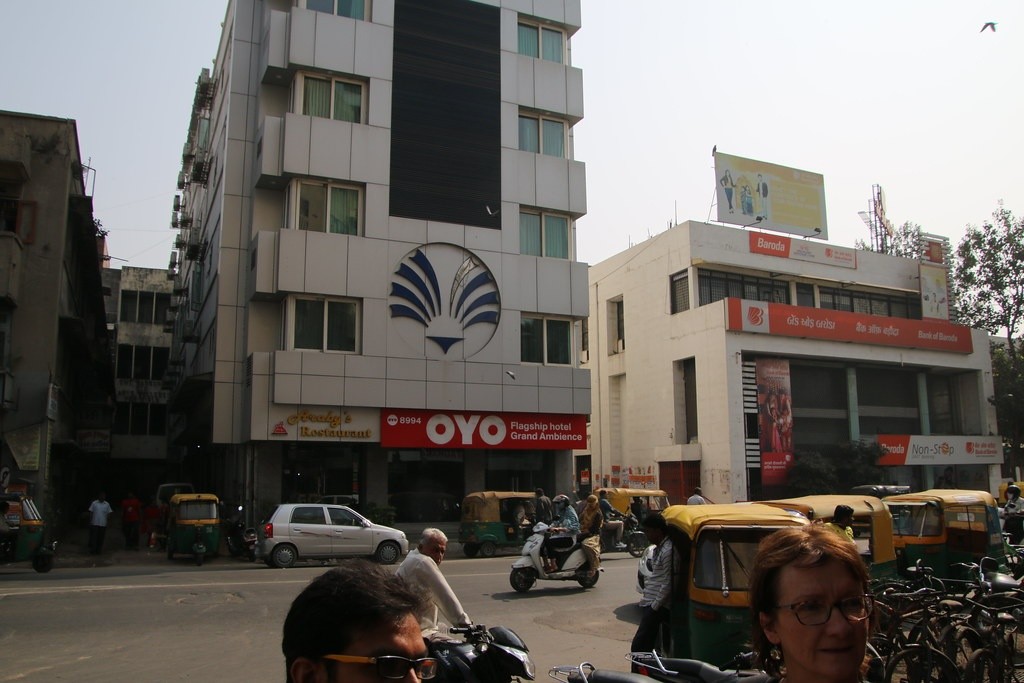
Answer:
[318,654,439,680]
[767,594,875,626]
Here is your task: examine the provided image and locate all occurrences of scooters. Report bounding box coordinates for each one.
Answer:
[508,513,604,593]
[425,624,537,683]
[596,504,650,558]
[545,641,785,683]
[220,504,258,563]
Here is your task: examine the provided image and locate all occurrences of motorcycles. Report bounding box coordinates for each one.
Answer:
[146,482,225,567]
[0,489,64,574]
[455,489,554,560]
[997,481,1024,516]
[592,486,672,552]
[655,500,816,673]
[880,487,1012,603]
[850,483,913,537]
[746,492,899,634]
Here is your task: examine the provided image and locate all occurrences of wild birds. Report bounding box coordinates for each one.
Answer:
[712,144,717,156]
[980,22,998,32]
[486,205,500,216]
[506,371,515,380]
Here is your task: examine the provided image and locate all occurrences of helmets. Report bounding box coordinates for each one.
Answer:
[553,495,569,509]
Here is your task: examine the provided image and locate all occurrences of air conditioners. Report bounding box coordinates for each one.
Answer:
[161,139,209,390]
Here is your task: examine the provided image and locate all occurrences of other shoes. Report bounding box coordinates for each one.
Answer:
[587,570,596,577]
[545,566,558,573]
[615,542,627,548]
[544,565,550,571]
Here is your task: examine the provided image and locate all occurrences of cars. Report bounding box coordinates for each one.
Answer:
[253,502,410,568]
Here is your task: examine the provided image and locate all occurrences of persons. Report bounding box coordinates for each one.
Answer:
[1004,481,1024,545]
[0,502,20,535]
[749,525,868,683]
[631,514,680,675]
[599,490,627,545]
[763,387,792,452]
[282,559,428,683]
[121,490,161,551]
[631,496,646,522]
[508,488,554,539]
[687,488,706,504]
[541,492,603,576]
[817,505,855,540]
[89,494,112,554]
[395,528,472,644]
[719,169,768,219]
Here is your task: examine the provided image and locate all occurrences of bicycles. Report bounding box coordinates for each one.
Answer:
[859,531,1024,683]
